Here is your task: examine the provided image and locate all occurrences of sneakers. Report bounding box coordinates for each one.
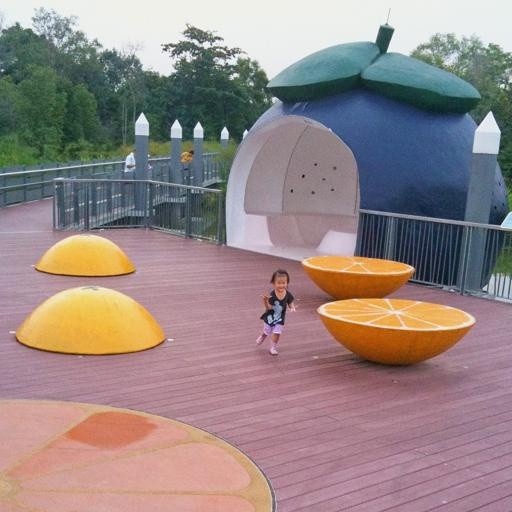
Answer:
[270,349,278,355]
[256,336,263,345]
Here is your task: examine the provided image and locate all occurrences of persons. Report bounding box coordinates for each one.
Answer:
[257,269,296,355]
[124,148,136,172]
[181,150,194,163]
